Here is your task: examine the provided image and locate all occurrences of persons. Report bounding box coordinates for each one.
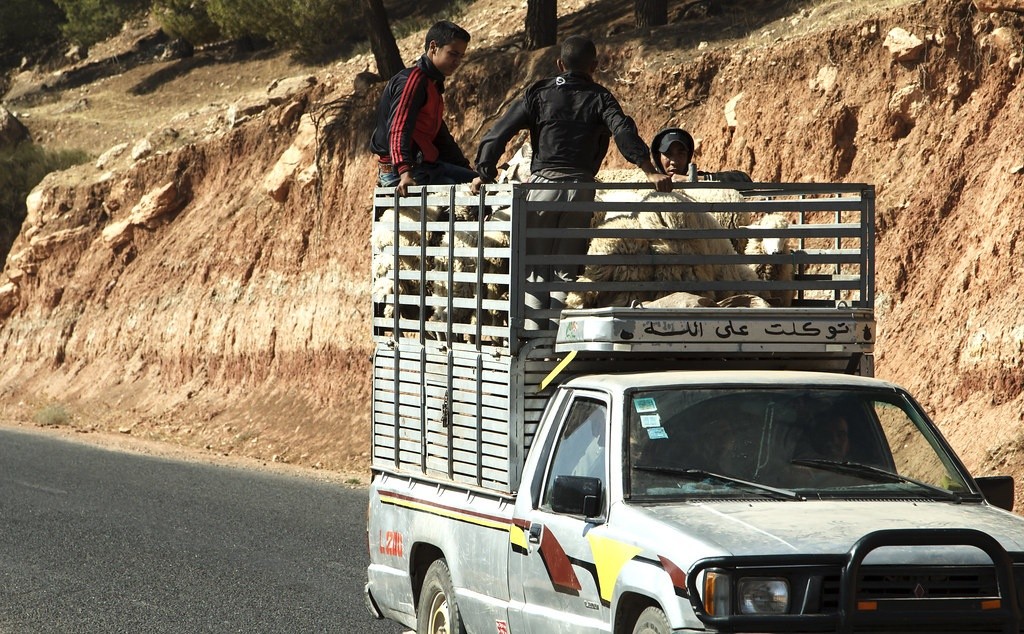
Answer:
[651,127,754,191]
[696,414,734,461]
[370,20,498,216]
[814,414,850,461]
[471,35,673,363]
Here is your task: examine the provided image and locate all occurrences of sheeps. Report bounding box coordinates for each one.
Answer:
[374,164,796,347]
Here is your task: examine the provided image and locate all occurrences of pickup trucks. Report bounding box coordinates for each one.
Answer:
[366,184,1024,634]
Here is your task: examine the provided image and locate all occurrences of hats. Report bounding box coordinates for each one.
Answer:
[657,132,688,153]
